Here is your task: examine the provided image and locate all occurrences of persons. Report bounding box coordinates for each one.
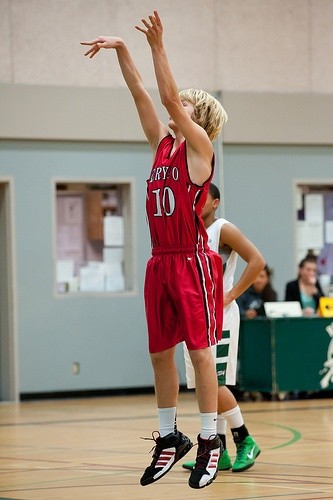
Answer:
[233,264,278,404]
[283,251,327,313]
[77,9,225,489]
[186,183,262,471]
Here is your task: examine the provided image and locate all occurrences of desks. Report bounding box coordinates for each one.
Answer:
[238,317,333,402]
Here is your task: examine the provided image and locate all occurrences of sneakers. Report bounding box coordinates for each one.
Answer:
[141,430,193,486]
[182,449,233,470]
[188,433,224,489]
[231,434,261,472]
